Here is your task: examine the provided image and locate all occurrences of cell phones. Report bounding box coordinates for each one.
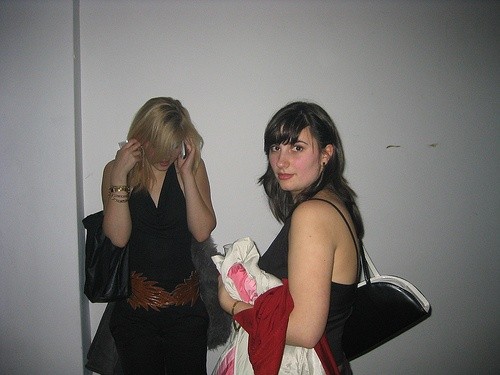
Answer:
[180,141,187,159]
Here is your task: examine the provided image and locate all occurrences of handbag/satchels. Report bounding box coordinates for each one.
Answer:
[292,197,432,363]
[82,211,130,303]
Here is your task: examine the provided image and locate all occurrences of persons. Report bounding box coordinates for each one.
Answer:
[85,97,232,375]
[218,101,364,375]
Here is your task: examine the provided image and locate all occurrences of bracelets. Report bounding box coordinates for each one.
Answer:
[108,185,129,203]
[232,301,241,331]
